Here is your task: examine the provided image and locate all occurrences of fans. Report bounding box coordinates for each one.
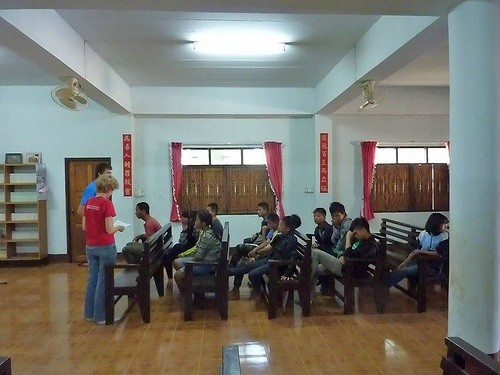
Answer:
[51,77,91,112]
[358,81,388,111]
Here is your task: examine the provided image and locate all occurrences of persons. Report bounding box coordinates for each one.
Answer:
[82,174,126,324]
[121,201,449,298]
[77,161,113,216]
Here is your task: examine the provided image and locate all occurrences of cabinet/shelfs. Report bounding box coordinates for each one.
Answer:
[0,164,48,261]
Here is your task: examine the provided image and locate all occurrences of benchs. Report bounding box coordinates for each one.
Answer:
[183,222,230,321]
[379,217,449,312]
[221,345,241,375]
[328,234,387,315]
[257,229,311,320]
[104,222,173,325]
[439,336,500,375]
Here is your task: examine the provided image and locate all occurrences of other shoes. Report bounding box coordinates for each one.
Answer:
[87,317,93,321]
[229,292,240,300]
[97,316,120,325]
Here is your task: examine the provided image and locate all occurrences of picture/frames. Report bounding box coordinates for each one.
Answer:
[5,153,23,164]
[26,153,42,164]
